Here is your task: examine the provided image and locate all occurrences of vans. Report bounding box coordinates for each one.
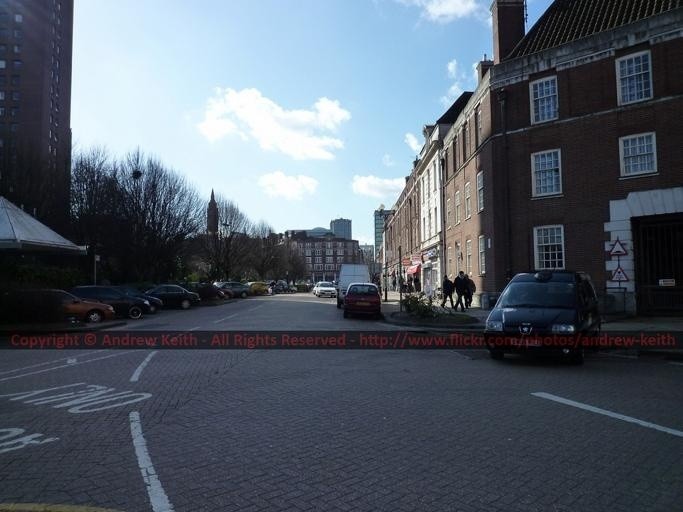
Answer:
[65,285,151,320]
[484,269,600,364]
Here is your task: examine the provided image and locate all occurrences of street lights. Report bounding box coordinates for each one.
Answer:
[358,247,371,264]
[377,204,395,304]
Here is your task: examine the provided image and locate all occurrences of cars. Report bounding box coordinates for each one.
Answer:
[178,282,225,303]
[213,281,250,300]
[243,282,269,296]
[114,286,163,315]
[143,284,201,310]
[265,279,338,295]
[315,281,337,298]
[21,288,116,324]
[342,282,383,320]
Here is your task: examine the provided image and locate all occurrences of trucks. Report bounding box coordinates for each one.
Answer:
[335,264,372,309]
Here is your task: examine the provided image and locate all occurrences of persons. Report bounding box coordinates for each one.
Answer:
[413,275,419,291]
[392,277,396,289]
[463,273,475,309]
[453,270,467,312]
[399,275,405,293]
[440,274,454,309]
[407,276,413,292]
